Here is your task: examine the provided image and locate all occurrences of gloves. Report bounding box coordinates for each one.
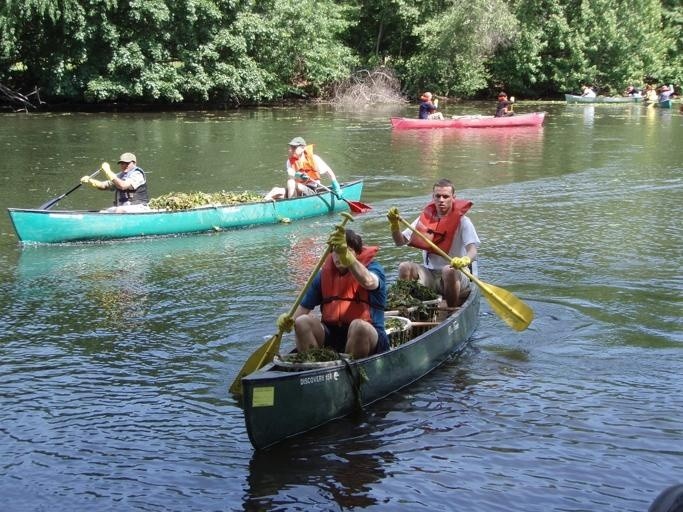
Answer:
[387,208,400,233]
[450,256,470,270]
[326,226,356,268]
[277,313,295,333]
[80,175,98,189]
[331,181,343,200]
[295,172,308,180]
[100,162,116,181]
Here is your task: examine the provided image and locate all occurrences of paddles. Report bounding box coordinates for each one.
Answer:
[394,213,533,332]
[229,211,353,395]
[38,168,101,210]
[305,174,372,213]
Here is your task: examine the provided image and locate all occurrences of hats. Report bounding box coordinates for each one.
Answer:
[329,230,362,253]
[117,153,137,165]
[662,86,670,91]
[627,86,633,93]
[287,137,307,146]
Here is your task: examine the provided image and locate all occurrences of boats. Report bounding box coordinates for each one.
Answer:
[4,176,368,249]
[238,253,480,461]
[564,92,647,104]
[388,111,550,128]
[654,99,673,109]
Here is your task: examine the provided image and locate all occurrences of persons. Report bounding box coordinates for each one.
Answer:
[386,178,481,318]
[578,80,675,105]
[80,152,149,213]
[277,225,390,359]
[419,91,445,120]
[262,137,344,200]
[494,91,517,117]
